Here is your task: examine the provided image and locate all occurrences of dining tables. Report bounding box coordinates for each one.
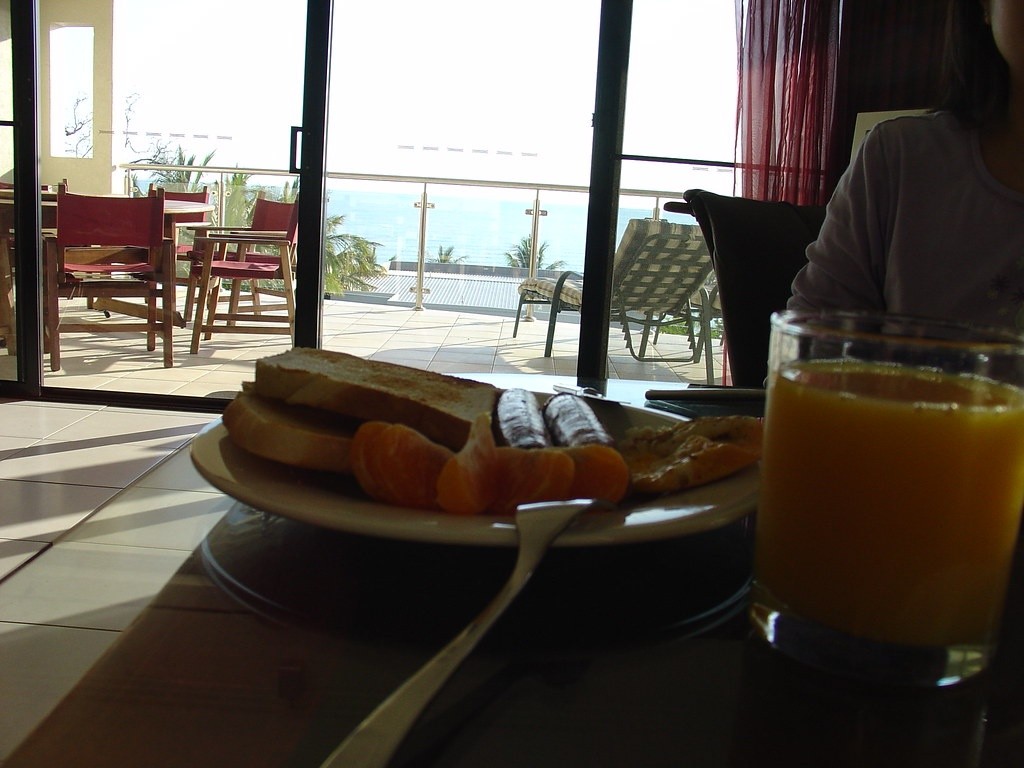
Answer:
[1,199,215,349]
[0,400,1024,768]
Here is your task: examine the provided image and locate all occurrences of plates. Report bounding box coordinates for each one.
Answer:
[191,390,758,545]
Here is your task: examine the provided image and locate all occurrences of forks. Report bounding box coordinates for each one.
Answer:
[318,498,616,768]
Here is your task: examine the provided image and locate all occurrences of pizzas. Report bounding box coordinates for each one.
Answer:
[622,413,768,494]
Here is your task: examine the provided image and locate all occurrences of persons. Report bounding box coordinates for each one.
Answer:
[786,0,1024,380]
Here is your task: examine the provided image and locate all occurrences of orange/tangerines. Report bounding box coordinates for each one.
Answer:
[351,422,628,517]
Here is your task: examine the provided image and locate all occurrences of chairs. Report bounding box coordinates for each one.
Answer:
[144,183,211,322]
[0,178,70,356]
[511,217,715,363]
[183,199,295,328]
[42,182,175,372]
[662,190,827,390]
[190,195,301,355]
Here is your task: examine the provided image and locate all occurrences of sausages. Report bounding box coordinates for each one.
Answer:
[540,392,618,448]
[494,388,549,449]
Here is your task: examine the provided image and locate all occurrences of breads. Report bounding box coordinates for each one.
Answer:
[254,348,496,452]
[224,380,361,473]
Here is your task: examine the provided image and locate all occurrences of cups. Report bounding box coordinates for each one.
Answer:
[745,310,1024,687]
